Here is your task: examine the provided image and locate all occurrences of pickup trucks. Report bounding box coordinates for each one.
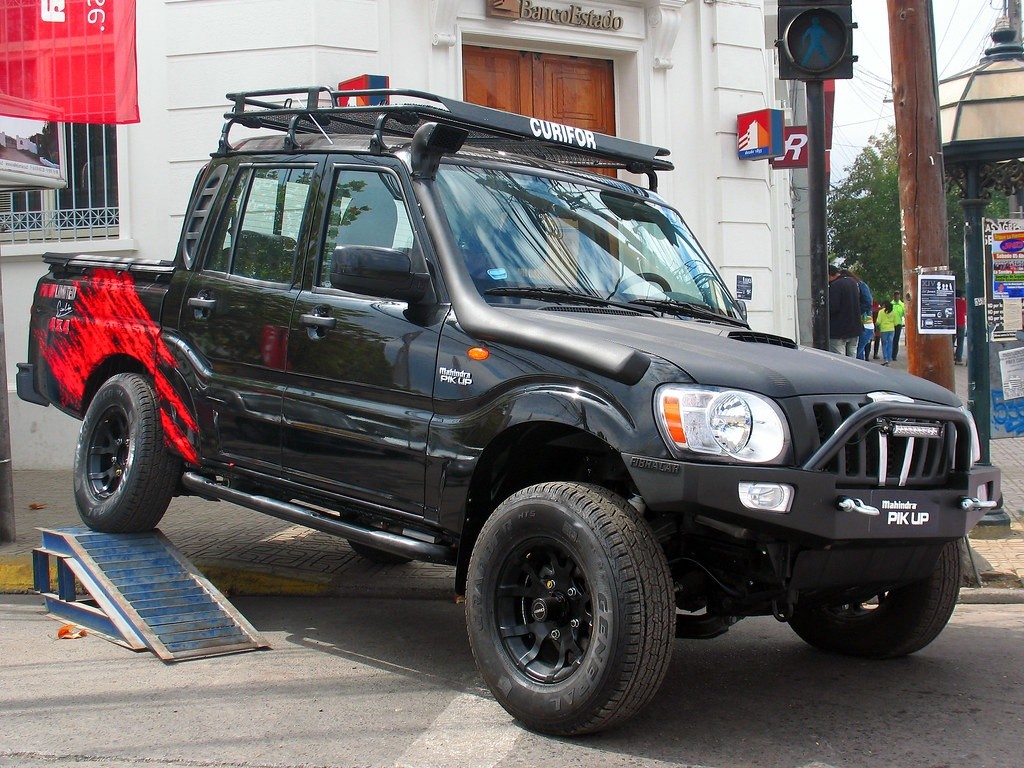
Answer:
[16,87,1009,740]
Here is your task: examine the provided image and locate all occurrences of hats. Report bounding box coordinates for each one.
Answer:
[894,290,900,294]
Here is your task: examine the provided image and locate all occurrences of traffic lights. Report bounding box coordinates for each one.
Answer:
[775,1,859,80]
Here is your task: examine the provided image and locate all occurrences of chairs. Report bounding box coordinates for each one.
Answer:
[334,186,398,248]
[498,207,547,288]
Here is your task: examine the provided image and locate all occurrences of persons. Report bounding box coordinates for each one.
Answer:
[994,260,1024,271]
[829,265,865,358]
[952,289,967,365]
[864,301,881,361]
[875,300,898,366]
[996,283,1004,292]
[841,270,874,361]
[891,291,905,361]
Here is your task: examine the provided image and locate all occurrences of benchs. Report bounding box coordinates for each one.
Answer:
[237,229,297,281]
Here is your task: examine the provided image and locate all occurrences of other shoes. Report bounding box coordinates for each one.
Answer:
[956,361,963,365]
[881,361,891,367]
[874,354,879,359]
